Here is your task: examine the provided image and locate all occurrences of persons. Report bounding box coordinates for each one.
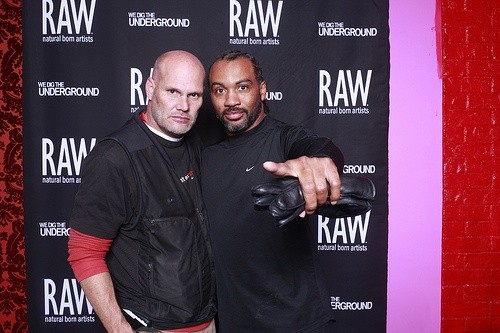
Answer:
[68,51,218,333]
[201,50,343,333]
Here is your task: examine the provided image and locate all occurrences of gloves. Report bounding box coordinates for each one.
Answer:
[250,174,375,228]
